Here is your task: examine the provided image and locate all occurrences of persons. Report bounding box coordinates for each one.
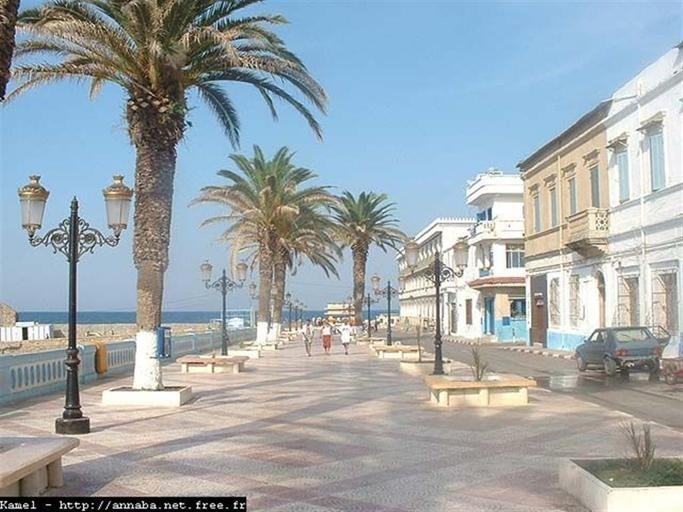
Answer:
[318,319,336,353]
[301,319,314,356]
[339,318,354,355]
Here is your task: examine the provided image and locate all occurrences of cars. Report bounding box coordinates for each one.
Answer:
[575,326,663,376]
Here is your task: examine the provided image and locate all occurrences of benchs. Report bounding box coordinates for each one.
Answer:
[0,435,81,497]
[174,354,249,374]
[374,342,537,409]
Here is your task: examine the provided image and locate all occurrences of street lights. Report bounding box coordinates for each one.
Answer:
[361,273,406,347]
[248,282,308,343]
[17,173,133,435]
[200,260,247,357]
[400,236,470,375]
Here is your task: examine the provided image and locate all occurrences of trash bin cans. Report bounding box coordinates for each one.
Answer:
[160,326,172,358]
